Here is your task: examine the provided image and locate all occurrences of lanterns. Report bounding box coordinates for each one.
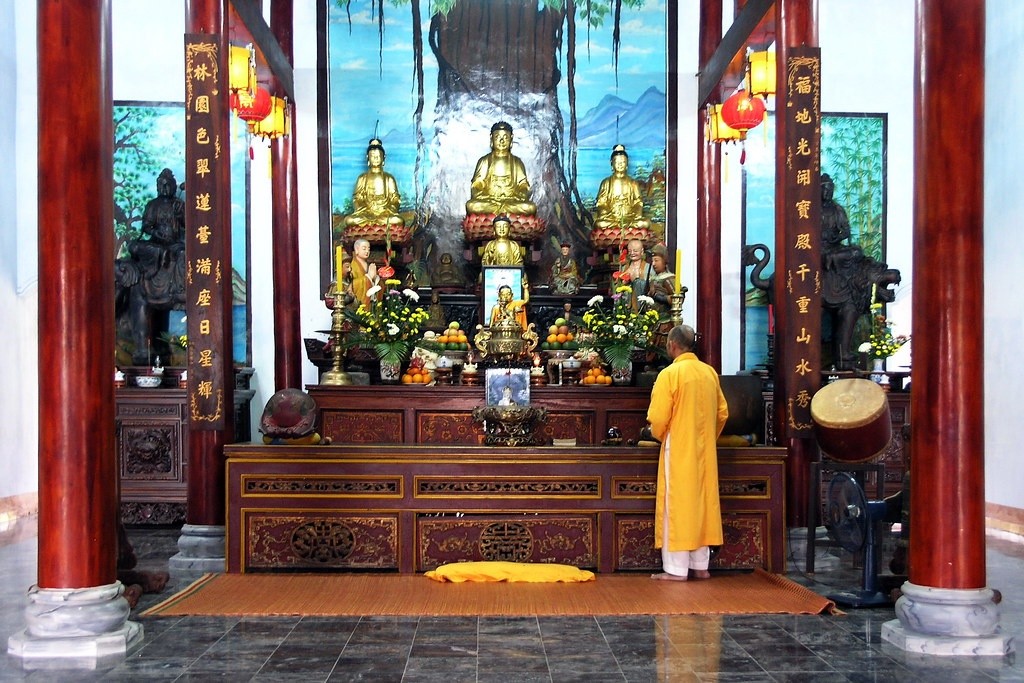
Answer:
[721,89,766,165]
[230,86,272,160]
[747,51,777,146]
[708,104,740,183]
[229,42,257,143]
[254,95,291,178]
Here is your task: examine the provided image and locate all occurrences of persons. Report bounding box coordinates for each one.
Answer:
[646,325,729,581]
[345,138,405,226]
[466,121,536,215]
[425,292,445,326]
[595,144,650,229]
[821,173,850,247]
[550,241,579,294]
[325,237,384,330]
[488,273,530,327]
[478,215,525,285]
[556,299,575,321]
[141,168,185,248]
[432,253,465,286]
[621,237,681,360]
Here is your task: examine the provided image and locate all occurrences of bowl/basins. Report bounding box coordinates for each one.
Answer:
[136,376,162,387]
[436,356,453,368]
[543,350,576,359]
[437,350,468,359]
[562,356,581,368]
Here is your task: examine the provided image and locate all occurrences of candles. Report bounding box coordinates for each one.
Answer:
[675,247,681,295]
[336,245,343,292]
[768,304,773,334]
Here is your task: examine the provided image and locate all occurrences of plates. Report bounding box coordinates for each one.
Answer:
[586,383,609,386]
[404,383,427,386]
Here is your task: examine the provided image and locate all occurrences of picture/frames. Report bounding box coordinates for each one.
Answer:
[481,265,524,328]
[741,112,888,368]
[316,0,678,300]
[112,98,252,367]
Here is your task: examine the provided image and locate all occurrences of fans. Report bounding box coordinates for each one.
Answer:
[826,473,894,609]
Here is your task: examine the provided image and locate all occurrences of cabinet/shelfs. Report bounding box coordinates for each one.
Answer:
[303,292,707,372]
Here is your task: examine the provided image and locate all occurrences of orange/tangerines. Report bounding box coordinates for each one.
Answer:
[583,368,613,384]
[437,335,468,350]
[401,367,432,383]
[541,333,579,350]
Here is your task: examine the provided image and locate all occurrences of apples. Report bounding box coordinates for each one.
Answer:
[443,321,465,336]
[549,317,569,334]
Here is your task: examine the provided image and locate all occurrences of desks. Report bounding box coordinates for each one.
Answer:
[305,384,656,444]
[221,439,791,578]
[114,364,256,525]
[749,366,913,526]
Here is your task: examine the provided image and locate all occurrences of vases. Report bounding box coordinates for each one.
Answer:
[609,360,634,386]
[377,354,400,384]
[871,357,883,386]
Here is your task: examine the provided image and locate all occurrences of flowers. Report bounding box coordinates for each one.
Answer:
[856,282,911,361]
[342,248,429,363]
[156,331,188,351]
[577,241,661,362]
